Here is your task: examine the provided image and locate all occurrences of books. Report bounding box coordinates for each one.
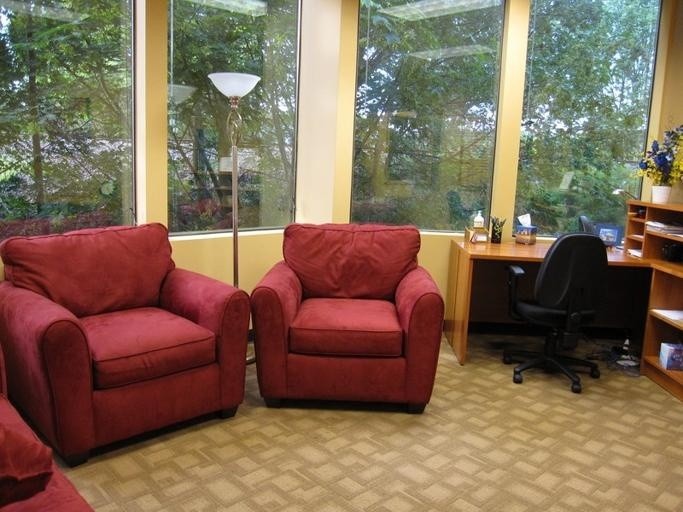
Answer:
[645,219,682,237]
[627,247,642,258]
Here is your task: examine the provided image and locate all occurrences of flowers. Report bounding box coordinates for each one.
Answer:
[629,124,683,186]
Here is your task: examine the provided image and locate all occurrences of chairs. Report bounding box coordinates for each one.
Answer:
[579,215,595,232]
[502,232,608,393]
[0,223,250,469]
[250,222,445,414]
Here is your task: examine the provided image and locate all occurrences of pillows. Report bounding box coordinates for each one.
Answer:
[0,392,54,480]
[283,223,420,299]
[0,222,175,316]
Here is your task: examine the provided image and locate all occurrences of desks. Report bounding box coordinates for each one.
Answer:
[444,239,649,366]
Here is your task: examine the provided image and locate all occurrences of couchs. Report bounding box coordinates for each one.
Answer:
[0,340,96,512]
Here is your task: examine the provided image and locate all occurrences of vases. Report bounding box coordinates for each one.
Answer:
[651,185,671,204]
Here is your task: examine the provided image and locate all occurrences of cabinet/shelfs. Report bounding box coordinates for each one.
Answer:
[623,198,683,402]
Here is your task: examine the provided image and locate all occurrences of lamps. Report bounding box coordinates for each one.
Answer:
[167,85,198,106]
[208,72,261,363]
